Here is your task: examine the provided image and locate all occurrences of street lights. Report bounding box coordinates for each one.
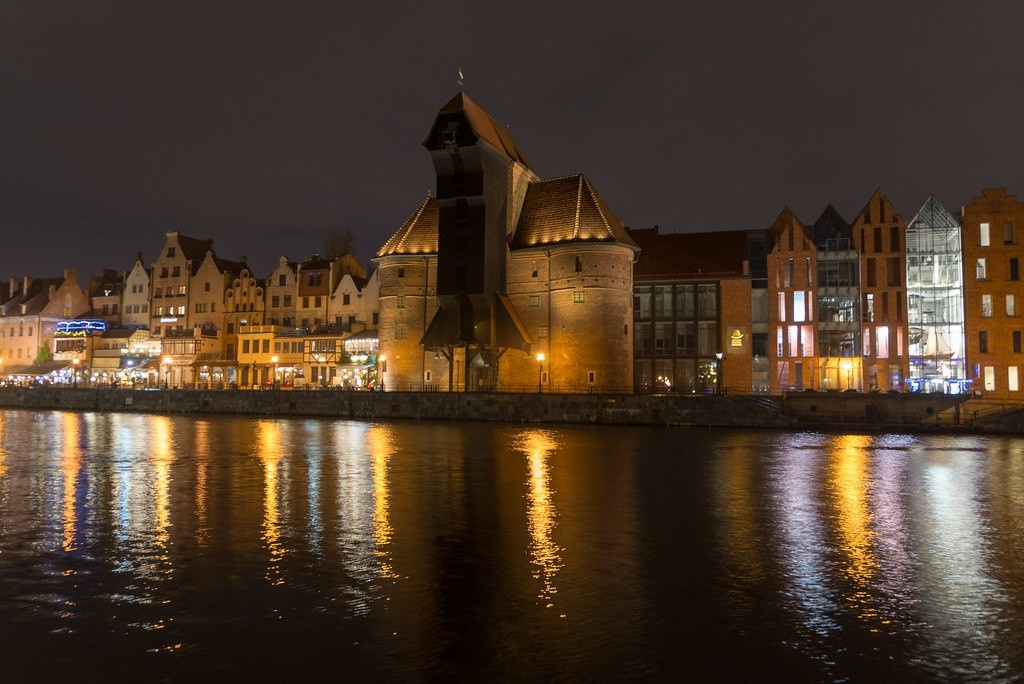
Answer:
[162,356,173,389]
[351,354,367,363]
[379,355,386,392]
[715,352,723,395]
[272,356,278,389]
[536,353,546,393]
[72,357,79,388]
